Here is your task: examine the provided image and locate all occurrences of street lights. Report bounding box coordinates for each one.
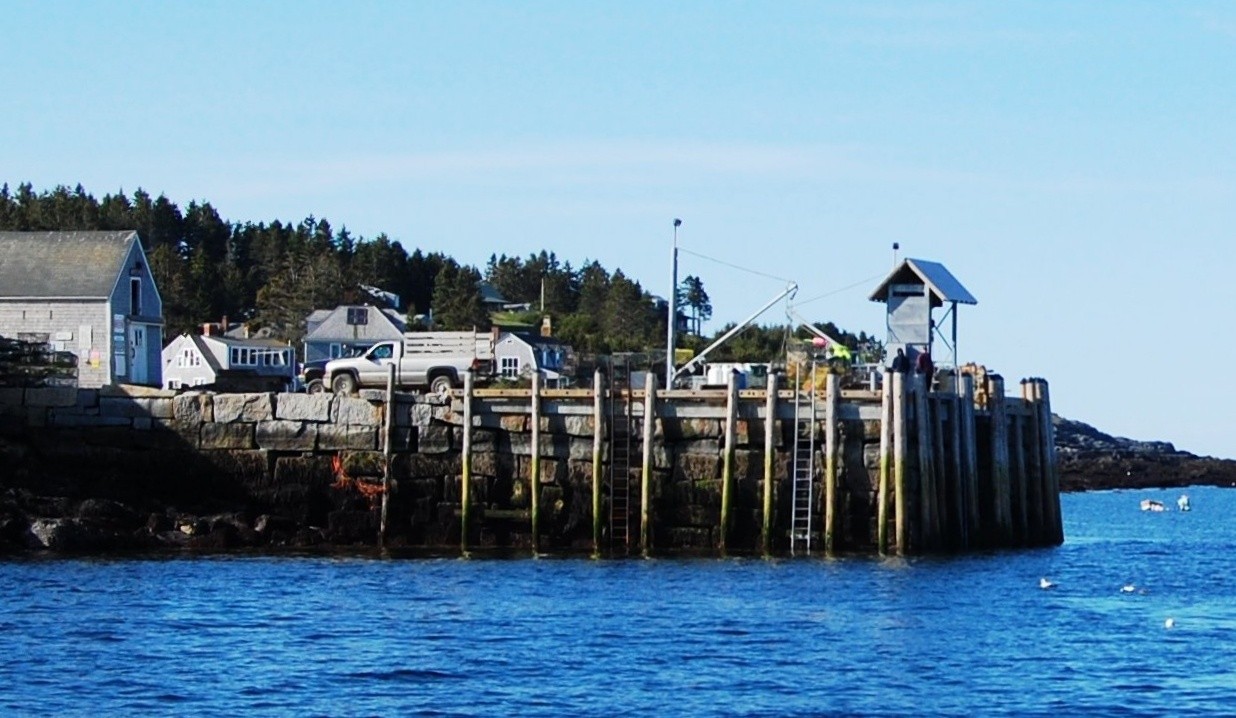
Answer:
[665,218,682,386]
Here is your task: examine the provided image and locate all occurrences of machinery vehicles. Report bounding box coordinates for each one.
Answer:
[675,285,800,388]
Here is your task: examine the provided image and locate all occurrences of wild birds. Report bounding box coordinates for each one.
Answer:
[1039,578,1058,589]
[1165,618,1174,628]
[1119,585,1136,593]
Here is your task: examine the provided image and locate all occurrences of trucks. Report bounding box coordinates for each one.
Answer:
[301,325,499,398]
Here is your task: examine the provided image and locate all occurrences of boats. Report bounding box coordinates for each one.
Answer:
[1176,495,1192,511]
[1139,501,1165,511]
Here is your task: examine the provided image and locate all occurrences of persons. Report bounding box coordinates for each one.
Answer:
[892,348,910,374]
[915,348,934,392]
